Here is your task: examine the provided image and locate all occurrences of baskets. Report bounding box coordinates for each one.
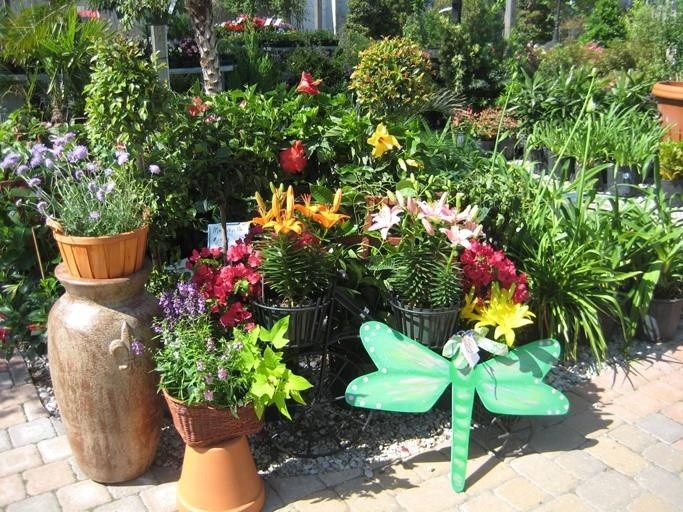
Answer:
[160,377,265,448]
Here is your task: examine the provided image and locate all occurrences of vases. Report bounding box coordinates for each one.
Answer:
[176,437,266,511]
[43,264,170,485]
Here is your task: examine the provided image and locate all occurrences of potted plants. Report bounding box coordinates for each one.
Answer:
[0,1,682,412]
